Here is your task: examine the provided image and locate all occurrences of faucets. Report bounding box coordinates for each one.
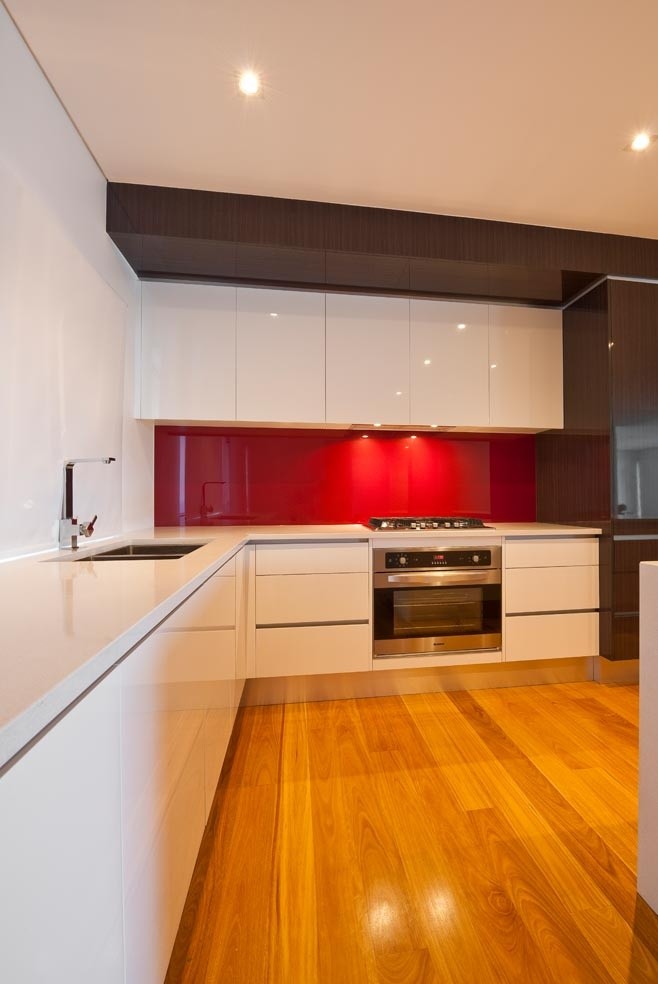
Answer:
[58,454,117,551]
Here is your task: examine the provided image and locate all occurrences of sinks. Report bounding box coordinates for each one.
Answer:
[93,542,207,556]
[73,555,184,562]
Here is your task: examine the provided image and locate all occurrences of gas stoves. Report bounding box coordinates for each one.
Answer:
[364,516,496,533]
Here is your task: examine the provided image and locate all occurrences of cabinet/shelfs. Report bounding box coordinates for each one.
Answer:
[503,536,601,662]
[255,543,373,677]
[134,278,564,435]
[1,550,250,984]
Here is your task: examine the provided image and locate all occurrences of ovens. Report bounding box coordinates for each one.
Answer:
[371,545,502,658]
